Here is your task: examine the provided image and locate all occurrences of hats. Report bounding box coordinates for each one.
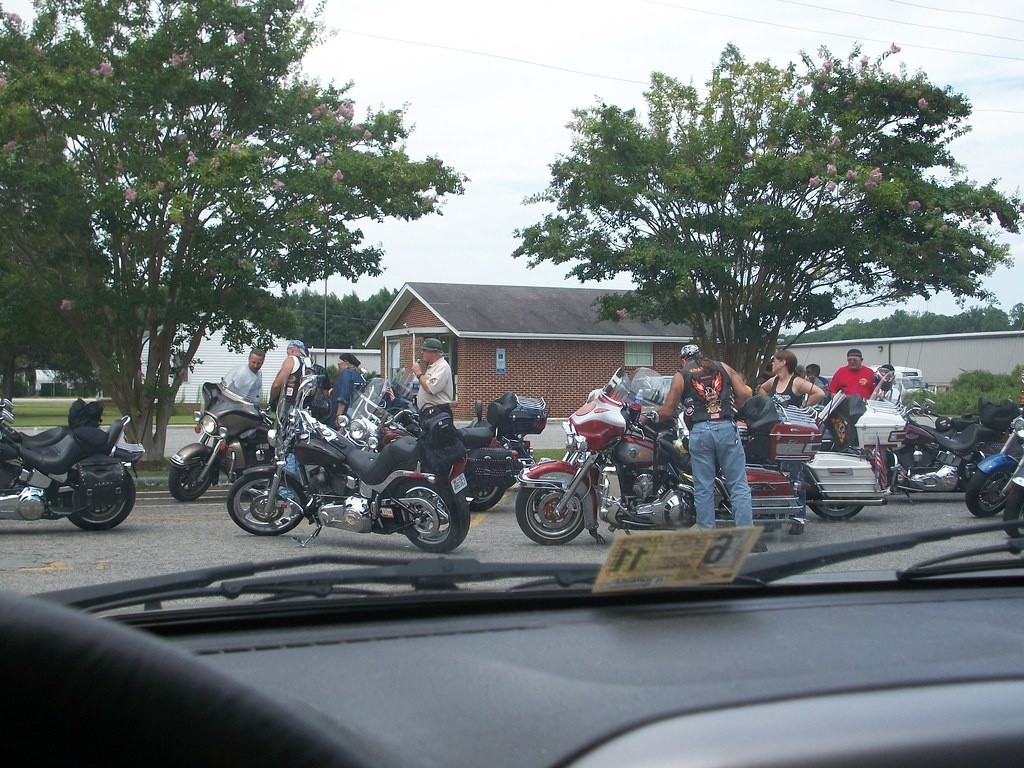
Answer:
[847,348,862,357]
[288,339,306,356]
[419,338,443,354]
[680,344,699,359]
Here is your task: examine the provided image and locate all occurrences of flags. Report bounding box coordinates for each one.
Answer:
[876,437,887,488]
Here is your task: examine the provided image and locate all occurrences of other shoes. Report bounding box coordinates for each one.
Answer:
[762,521,782,532]
[789,523,804,534]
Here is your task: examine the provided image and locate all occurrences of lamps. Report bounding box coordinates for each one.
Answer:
[878,345,883,352]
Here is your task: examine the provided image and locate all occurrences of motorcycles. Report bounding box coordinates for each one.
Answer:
[227,357,471,554]
[0,398,146,531]
[513,364,825,551]
[166,379,550,513]
[626,365,1024,540]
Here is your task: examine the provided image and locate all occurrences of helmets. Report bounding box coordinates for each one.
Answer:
[304,397,330,422]
[874,367,893,390]
[935,416,952,432]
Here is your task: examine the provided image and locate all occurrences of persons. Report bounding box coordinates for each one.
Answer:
[733,349,903,534]
[411,338,453,425]
[328,353,365,432]
[269,340,313,422]
[638,344,768,553]
[222,348,265,404]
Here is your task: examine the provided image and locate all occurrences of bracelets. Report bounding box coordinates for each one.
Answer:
[416,374,423,379]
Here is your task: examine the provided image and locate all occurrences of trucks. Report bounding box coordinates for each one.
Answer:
[869,366,928,393]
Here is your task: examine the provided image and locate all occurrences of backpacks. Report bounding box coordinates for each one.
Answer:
[420,402,456,449]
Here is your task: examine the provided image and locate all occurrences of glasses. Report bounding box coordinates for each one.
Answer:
[338,362,345,366]
[848,358,860,362]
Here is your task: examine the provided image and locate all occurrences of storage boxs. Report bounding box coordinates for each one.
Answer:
[854,402,907,451]
[768,406,822,461]
[979,400,1021,434]
[805,451,881,497]
[507,397,547,434]
